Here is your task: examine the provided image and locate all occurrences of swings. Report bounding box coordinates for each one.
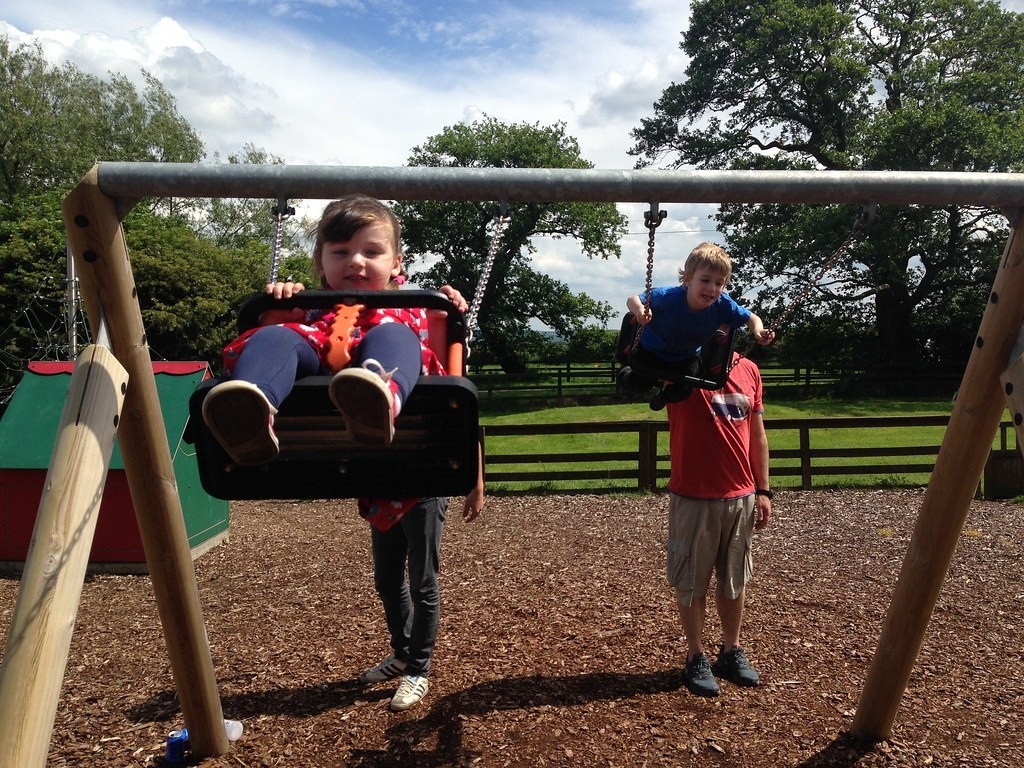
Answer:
[616,205,878,390]
[184,199,513,502]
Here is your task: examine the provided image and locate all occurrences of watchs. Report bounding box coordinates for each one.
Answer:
[755,488,774,499]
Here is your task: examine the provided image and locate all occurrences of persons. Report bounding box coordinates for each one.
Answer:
[666,350,774,697]
[616,242,775,412]
[202,195,469,467]
[358,439,483,710]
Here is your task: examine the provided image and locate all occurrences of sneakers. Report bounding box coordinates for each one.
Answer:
[366,653,409,682]
[717,645,760,685]
[329,359,399,446]
[650,381,673,411]
[202,381,280,466]
[618,366,631,376]
[390,675,429,709]
[682,653,719,694]
